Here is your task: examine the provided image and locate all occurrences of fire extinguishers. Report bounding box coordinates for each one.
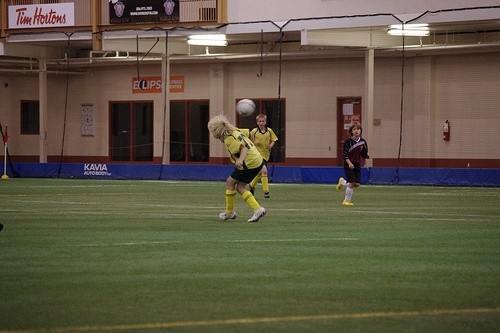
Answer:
[442,120,450,140]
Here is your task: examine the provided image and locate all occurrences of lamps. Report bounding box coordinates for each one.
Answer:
[187,39,228,47]
[389,23,429,29]
[386,29,431,36]
[187,34,226,40]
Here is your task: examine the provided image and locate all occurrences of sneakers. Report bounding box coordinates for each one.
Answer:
[342,200,354,206]
[246,207,266,223]
[219,211,237,220]
[336,177,344,192]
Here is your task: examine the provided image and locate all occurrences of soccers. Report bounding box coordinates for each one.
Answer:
[236,98,256,117]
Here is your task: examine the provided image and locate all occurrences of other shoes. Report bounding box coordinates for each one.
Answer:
[249,183,255,196]
[264,192,270,198]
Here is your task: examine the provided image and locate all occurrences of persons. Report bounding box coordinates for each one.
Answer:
[249,114,278,199]
[208,115,266,222]
[336,124,370,206]
